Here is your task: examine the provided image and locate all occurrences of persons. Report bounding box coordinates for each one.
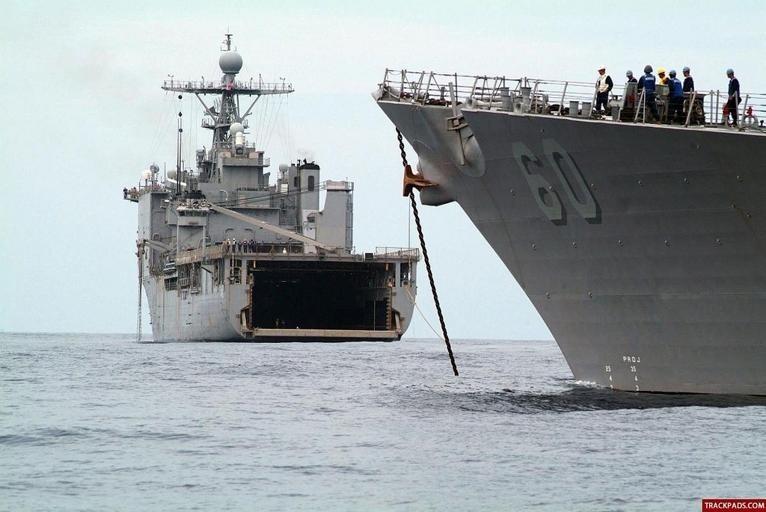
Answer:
[626,70,638,83]
[662,69,685,125]
[682,67,694,92]
[636,65,661,125]
[657,66,668,84]
[718,68,741,126]
[595,66,614,111]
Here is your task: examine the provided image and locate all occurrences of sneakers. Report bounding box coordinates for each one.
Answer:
[650,119,685,125]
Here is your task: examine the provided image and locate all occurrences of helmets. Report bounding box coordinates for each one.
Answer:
[644,65,653,72]
[626,70,633,77]
[658,68,665,74]
[669,70,676,75]
[597,65,606,71]
[683,67,690,72]
[727,69,734,74]
